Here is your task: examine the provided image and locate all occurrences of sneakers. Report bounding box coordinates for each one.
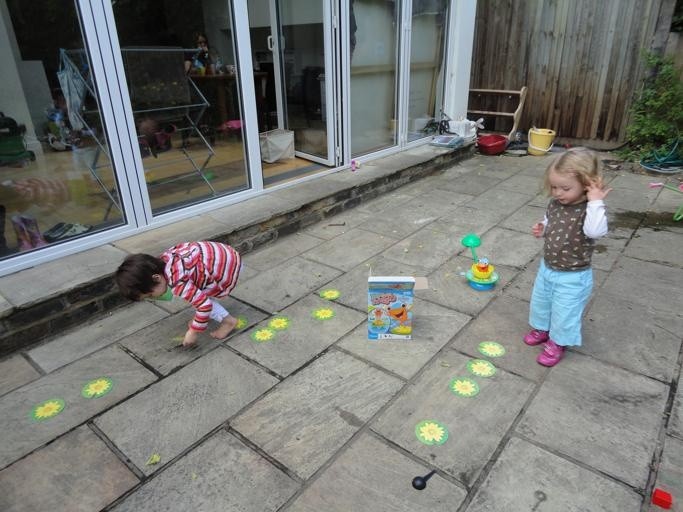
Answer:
[538,340,565,367]
[525,329,549,346]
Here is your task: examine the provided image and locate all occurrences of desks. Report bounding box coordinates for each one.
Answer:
[191,71,267,146]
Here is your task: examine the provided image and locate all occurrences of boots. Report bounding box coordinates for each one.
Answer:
[11,214,44,252]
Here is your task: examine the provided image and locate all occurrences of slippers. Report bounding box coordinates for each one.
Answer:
[44,221,92,242]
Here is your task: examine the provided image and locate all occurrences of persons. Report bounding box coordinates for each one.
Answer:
[517,146,615,369]
[181,33,210,75]
[115,239,243,349]
[0,176,72,232]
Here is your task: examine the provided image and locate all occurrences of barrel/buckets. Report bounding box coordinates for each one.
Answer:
[527,127,557,155]
